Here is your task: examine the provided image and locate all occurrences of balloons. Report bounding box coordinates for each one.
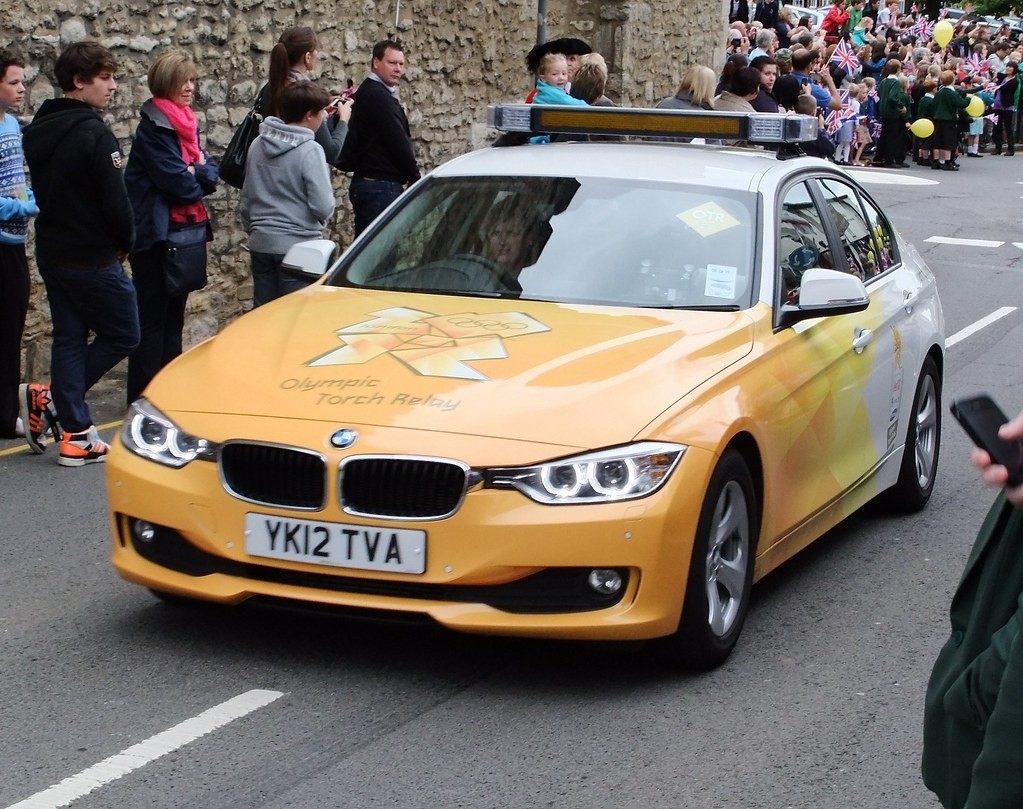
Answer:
[867,225,883,260]
[966,95,985,117]
[908,118,935,138]
[933,21,954,48]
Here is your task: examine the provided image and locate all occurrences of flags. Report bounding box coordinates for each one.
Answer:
[869,119,883,140]
[963,52,983,76]
[907,19,932,43]
[831,39,861,76]
[824,94,867,137]
[850,131,860,149]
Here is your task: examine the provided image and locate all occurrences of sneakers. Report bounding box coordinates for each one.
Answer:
[19,383,58,454]
[57,425,110,467]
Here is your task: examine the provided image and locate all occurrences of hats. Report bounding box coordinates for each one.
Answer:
[526,33,593,76]
[774,48,792,63]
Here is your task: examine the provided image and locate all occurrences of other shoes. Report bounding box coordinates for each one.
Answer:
[872,153,961,171]
[967,152,973,156]
[991,148,1002,155]
[834,160,842,165]
[972,153,984,157]
[1004,149,1014,156]
[15,417,54,438]
[853,161,865,166]
[843,161,853,166]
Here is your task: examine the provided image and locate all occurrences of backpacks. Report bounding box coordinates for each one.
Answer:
[324,78,380,173]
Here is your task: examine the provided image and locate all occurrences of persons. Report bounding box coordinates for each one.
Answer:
[17,41,142,468]
[492,36,626,148]
[480,194,538,281]
[848,241,896,282]
[921,411,1022,809]
[640,0,1023,171]
[239,79,334,311]
[0,47,41,441]
[125,52,218,408]
[258,26,353,166]
[345,40,427,241]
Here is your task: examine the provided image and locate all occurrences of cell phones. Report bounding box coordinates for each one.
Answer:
[802,77,807,90]
[332,98,348,109]
[810,72,822,80]
[731,39,741,47]
[950,393,1023,488]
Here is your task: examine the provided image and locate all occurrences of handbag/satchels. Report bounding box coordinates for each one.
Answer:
[162,222,207,296]
[219,81,270,189]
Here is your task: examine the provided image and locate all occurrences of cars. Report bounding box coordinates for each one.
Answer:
[781,3,836,33]
[932,9,1023,52]
[99,105,948,668]
